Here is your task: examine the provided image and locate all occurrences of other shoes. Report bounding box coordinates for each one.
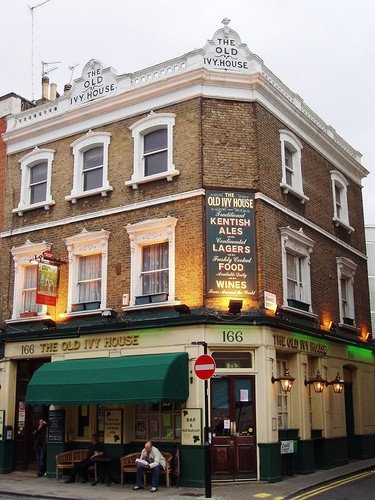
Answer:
[133,483,143,490]
[36,471,43,476]
[150,486,157,492]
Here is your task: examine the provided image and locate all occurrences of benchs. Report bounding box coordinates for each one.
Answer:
[55,449,97,484]
[119,452,172,488]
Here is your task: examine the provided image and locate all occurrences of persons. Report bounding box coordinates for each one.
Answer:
[64,433,104,483]
[31,419,52,477]
[132,441,166,492]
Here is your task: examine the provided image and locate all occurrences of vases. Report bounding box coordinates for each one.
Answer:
[20,311,37,319]
[287,299,310,312]
[343,317,354,325]
[71,300,101,312]
[135,292,169,305]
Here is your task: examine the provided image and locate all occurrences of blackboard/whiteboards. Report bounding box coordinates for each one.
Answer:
[46,409,65,444]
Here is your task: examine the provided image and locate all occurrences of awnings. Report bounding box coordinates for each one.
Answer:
[25,351,189,406]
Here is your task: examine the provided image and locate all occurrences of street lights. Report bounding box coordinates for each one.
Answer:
[190,341,211,498]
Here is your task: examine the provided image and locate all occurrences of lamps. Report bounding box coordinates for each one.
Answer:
[173,304,193,316]
[271,366,295,392]
[304,369,327,393]
[275,308,286,319]
[102,310,119,323]
[366,332,373,343]
[41,318,57,330]
[325,371,347,393]
[228,299,243,316]
[328,321,339,334]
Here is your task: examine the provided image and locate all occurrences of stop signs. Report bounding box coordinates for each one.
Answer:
[194,356,216,380]
